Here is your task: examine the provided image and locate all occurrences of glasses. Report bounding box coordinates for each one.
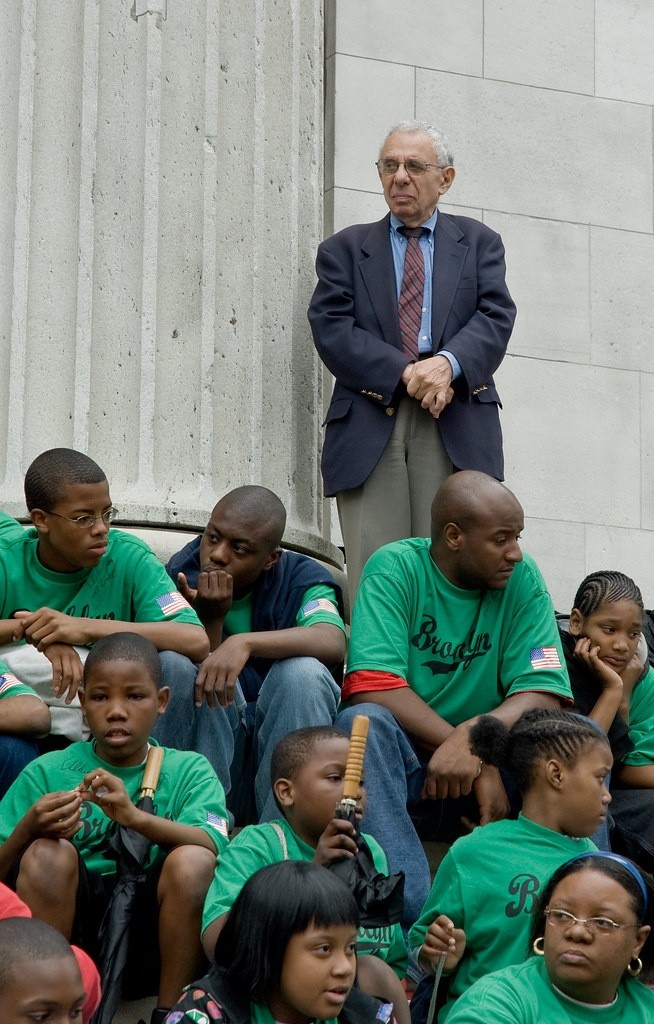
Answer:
[543,909,641,936]
[375,158,442,176]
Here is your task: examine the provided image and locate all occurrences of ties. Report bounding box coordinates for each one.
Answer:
[396,226,429,363]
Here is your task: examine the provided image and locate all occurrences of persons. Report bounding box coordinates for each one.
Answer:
[1,447,654,1024]
[307,119,518,616]
